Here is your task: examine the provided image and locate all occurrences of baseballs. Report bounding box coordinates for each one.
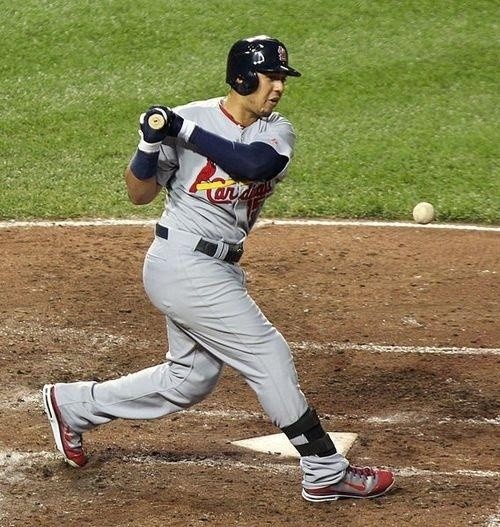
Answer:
[413,202,434,224]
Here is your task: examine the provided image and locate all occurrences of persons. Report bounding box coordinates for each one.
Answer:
[41,35,396,504]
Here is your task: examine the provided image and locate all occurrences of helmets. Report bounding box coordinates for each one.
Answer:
[226,34,301,96]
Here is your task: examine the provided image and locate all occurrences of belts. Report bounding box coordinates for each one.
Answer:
[156,222,244,262]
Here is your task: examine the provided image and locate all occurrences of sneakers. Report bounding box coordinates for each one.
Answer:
[42,383,87,468]
[301,465,396,503]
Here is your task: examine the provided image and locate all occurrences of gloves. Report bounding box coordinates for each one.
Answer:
[149,105,196,144]
[137,105,175,154]
